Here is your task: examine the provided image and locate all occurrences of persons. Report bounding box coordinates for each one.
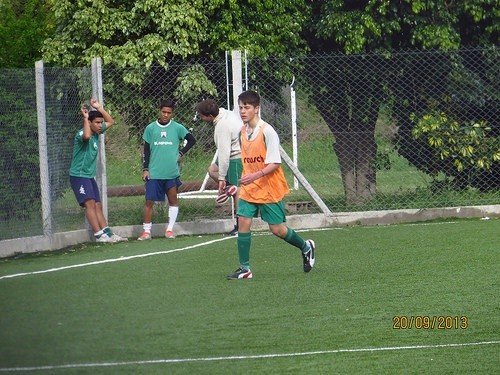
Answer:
[227,90,316,282]
[137,96,196,241]
[196,99,245,237]
[68,99,130,243]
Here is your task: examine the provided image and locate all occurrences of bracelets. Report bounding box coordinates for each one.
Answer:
[143,168,148,171]
[179,152,183,156]
[260,170,266,178]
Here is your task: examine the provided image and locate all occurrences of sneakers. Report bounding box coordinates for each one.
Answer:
[302,239,316,273]
[227,268,253,279]
[96,234,116,243]
[137,231,152,241]
[165,230,175,239]
[110,233,128,242]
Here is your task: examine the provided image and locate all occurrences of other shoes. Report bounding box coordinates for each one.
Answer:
[228,228,238,236]
[216,185,238,204]
